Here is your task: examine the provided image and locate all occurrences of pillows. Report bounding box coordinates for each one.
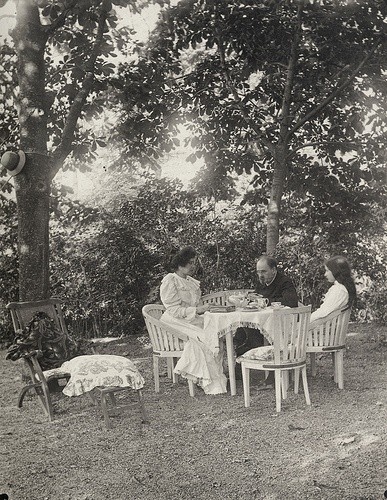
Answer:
[60,351,145,399]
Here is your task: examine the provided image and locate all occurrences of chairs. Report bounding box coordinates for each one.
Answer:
[235,303,313,413]
[200,289,254,306]
[293,303,352,394]
[4,297,97,420]
[142,303,196,397]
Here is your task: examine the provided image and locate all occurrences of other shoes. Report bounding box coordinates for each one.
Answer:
[226,370,242,380]
[253,383,273,390]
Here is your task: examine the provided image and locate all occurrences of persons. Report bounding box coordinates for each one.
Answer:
[222,254,298,379]
[306,254,357,350]
[158,246,228,395]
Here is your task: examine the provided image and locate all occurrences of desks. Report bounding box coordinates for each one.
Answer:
[202,305,296,396]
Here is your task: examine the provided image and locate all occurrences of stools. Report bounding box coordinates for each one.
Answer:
[94,387,147,431]
